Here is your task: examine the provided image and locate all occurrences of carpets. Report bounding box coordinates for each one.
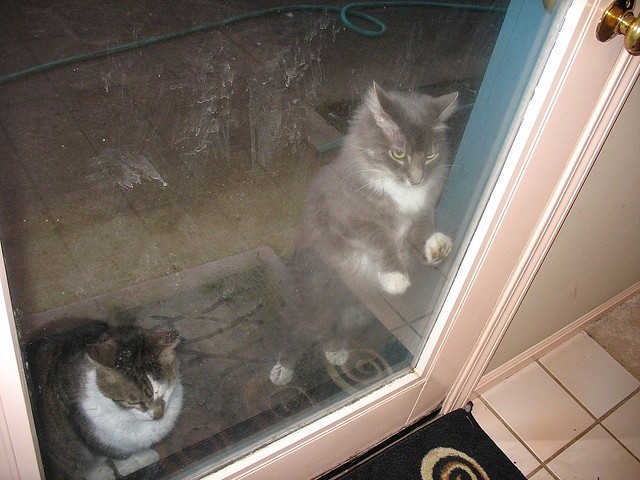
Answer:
[325,406,530,480]
[15,247,416,480]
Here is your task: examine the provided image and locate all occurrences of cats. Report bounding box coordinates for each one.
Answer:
[267,79,461,388]
[22,314,188,480]
